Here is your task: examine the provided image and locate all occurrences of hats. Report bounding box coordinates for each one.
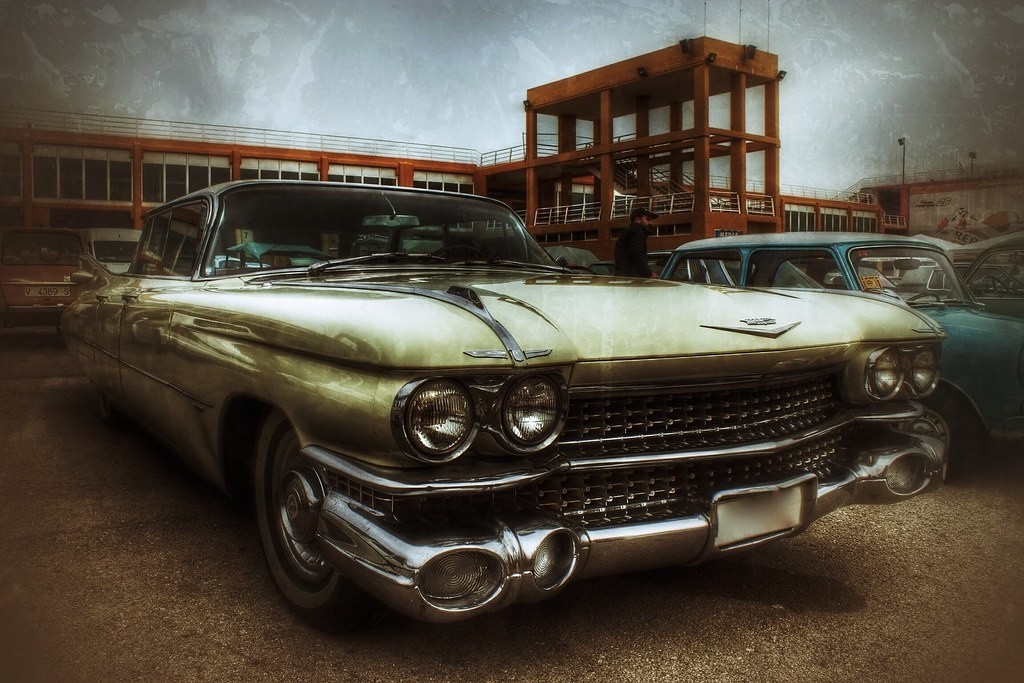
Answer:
[630,208,657,223]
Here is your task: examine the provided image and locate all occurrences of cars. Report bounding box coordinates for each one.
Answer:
[946,231,1024,318]
[658,230,1024,444]
[80,228,144,275]
[60,178,953,629]
[0,226,89,313]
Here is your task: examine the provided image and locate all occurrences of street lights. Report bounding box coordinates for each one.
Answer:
[897,136,905,184]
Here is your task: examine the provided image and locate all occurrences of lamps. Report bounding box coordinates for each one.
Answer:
[637,67,645,76]
[743,45,757,59]
[778,70,787,80]
[680,39,693,54]
[707,53,717,62]
[523,100,530,106]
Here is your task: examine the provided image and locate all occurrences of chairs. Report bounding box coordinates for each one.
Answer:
[401,239,444,256]
[244,254,292,268]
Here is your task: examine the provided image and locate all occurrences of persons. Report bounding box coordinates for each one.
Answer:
[615,208,659,279]
